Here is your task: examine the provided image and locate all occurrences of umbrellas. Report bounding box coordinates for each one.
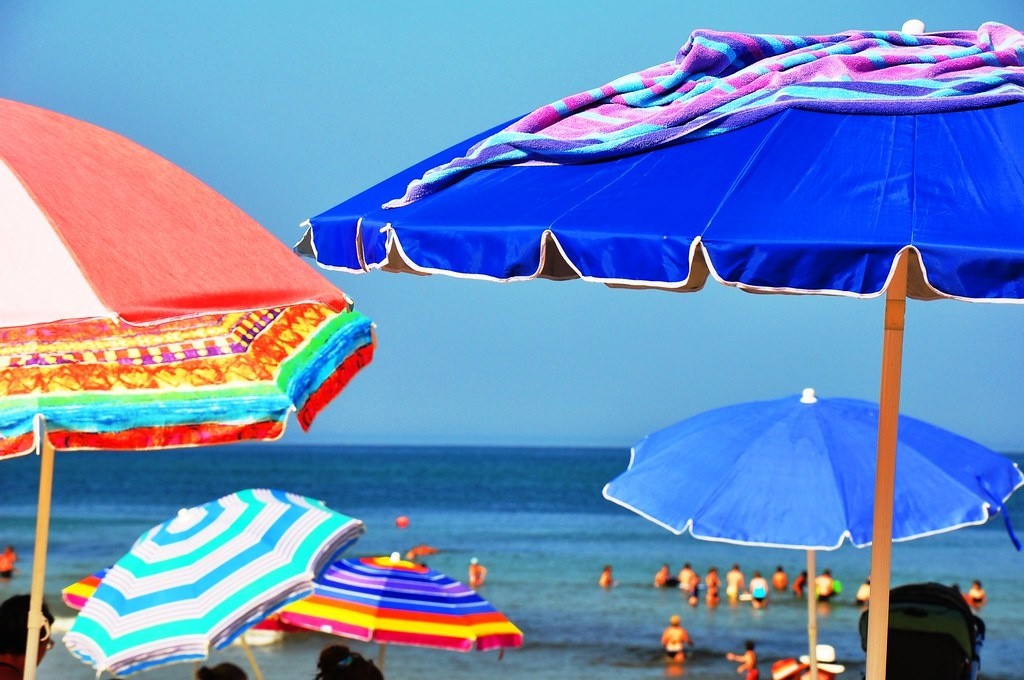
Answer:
[275,552,523,669]
[296,14,1024,680]
[0,302,376,680]
[60,487,369,680]
[60,564,310,680]
[602,388,1024,680]
[1,98,356,334]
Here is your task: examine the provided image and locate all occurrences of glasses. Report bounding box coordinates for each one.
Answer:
[335,653,360,670]
[44,639,55,650]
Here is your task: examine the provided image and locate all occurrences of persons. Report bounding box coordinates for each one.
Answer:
[771,643,845,680]
[468,556,487,589]
[857,581,985,680]
[856,576,872,610]
[0,593,56,680]
[661,615,694,661]
[652,562,770,609]
[196,662,247,680]
[772,565,836,602]
[313,644,385,680]
[598,565,614,588]
[968,580,987,608]
[726,639,760,680]
[0,544,17,578]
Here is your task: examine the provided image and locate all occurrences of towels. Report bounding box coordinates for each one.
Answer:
[377,16,1024,209]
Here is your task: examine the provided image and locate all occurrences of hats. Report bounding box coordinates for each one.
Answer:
[799,644,845,674]
[772,660,809,680]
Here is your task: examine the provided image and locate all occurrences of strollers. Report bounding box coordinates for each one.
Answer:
[856,579,988,680]
